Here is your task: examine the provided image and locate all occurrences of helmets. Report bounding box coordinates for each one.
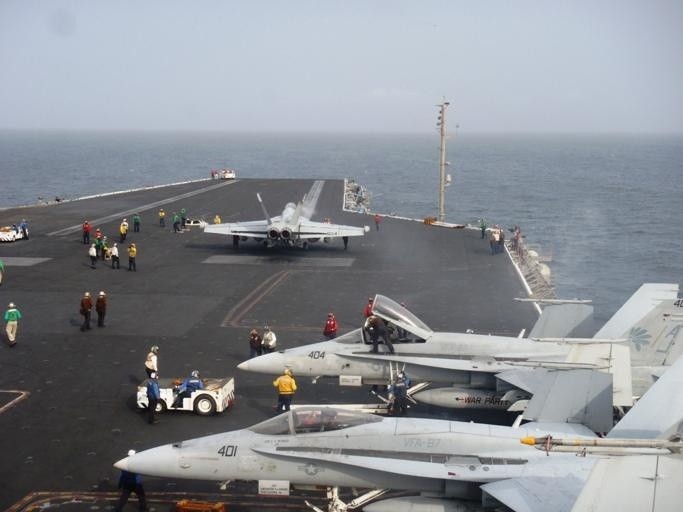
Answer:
[151,345,160,355]
[8,302,14,309]
[150,371,159,379]
[98,291,106,296]
[368,298,373,303]
[284,368,293,376]
[264,326,269,332]
[328,313,334,320]
[191,369,199,378]
[85,292,90,297]
[83,207,185,253]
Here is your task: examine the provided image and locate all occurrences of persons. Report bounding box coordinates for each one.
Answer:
[96,291,108,328]
[145,297,410,425]
[233,235,240,250]
[479,218,520,255]
[213,214,222,224]
[80,291,94,332]
[374,214,382,231]
[210,167,234,179]
[83,212,140,272]
[158,208,187,234]
[322,217,333,223]
[13,223,19,234]
[0,260,5,286]
[113,448,148,512]
[21,218,30,241]
[3,301,23,347]
[342,236,348,250]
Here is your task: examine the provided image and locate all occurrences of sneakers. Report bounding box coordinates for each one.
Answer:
[9,340,17,347]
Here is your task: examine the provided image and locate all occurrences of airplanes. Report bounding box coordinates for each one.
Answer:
[200,179,369,249]
[235,283,683,413]
[112,358,682,512]
